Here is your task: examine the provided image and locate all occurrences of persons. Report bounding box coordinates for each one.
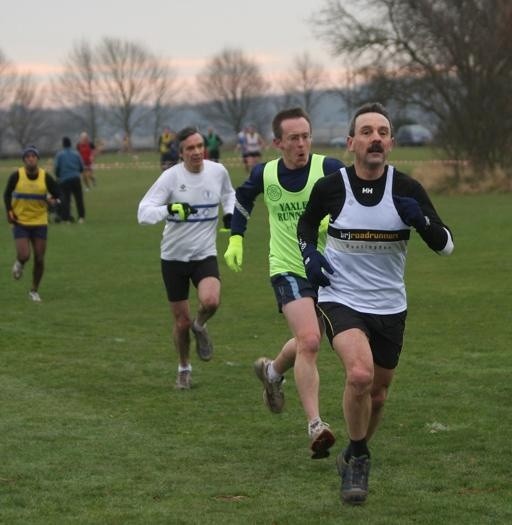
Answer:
[158,125,265,177]
[224,107,347,462]
[53,137,85,224]
[76,132,98,190]
[137,127,237,393]
[296,103,456,507]
[3,144,64,303]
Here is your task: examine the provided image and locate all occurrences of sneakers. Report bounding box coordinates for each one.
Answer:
[11,257,26,281]
[340,445,374,505]
[308,420,337,461]
[174,365,195,391]
[28,289,44,303]
[189,317,216,363]
[251,355,289,415]
[334,447,347,479]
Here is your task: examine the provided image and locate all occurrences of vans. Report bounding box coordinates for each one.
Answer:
[396,123,434,145]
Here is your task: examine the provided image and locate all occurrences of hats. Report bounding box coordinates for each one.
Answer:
[22,144,41,163]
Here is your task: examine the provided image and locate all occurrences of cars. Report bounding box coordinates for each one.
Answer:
[330,133,351,147]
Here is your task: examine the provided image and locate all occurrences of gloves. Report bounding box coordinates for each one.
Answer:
[392,193,426,228]
[218,212,238,233]
[168,201,200,223]
[223,232,246,275]
[299,248,337,288]
